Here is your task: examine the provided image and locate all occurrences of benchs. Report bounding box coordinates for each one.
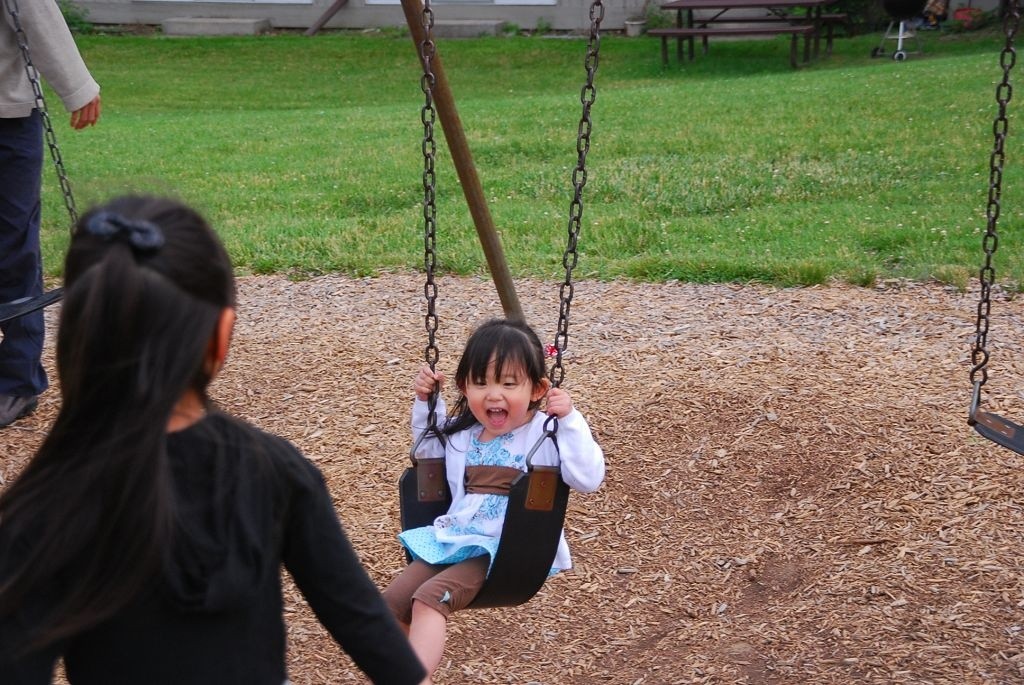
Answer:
[694,13,848,53]
[647,25,815,67]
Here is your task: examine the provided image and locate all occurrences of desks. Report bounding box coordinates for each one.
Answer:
[659,0,824,60]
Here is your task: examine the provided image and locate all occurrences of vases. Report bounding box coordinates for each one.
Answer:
[623,18,647,37]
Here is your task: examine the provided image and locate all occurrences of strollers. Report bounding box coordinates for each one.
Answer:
[870,0,950,63]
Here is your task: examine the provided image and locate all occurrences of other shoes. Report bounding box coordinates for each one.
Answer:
[0,391,39,430]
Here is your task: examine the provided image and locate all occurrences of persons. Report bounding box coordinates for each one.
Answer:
[0,195,428,685]
[382,319,605,685]
[0,0,101,425]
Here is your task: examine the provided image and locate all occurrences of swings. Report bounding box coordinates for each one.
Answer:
[398,0,606,610]
[0,0,80,322]
[967,0,1024,457]
